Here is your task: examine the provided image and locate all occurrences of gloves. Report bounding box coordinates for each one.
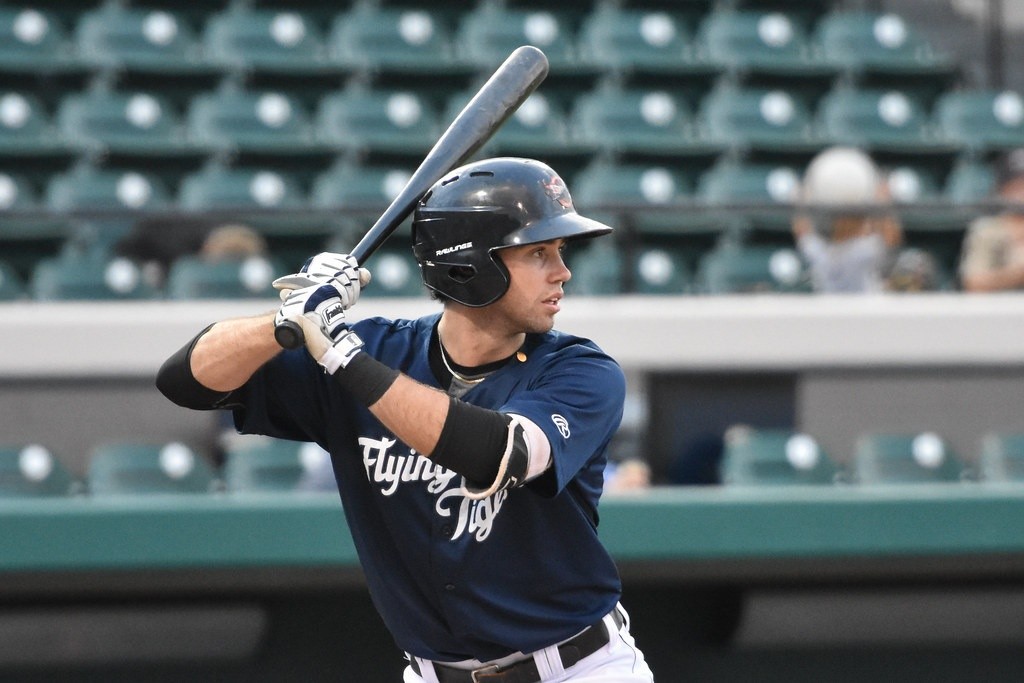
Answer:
[273,250,371,374]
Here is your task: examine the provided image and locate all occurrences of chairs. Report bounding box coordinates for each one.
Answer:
[0,0,1024,502]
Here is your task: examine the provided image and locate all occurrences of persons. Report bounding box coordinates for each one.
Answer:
[787,187,898,292]
[156,157,653,683]
[958,148,1024,291]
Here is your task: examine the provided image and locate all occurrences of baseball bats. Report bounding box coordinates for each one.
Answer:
[273,44,549,350]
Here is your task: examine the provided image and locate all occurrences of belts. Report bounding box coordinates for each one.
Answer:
[411,606,623,683]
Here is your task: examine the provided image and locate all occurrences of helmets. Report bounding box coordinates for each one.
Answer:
[410,156,613,308]
